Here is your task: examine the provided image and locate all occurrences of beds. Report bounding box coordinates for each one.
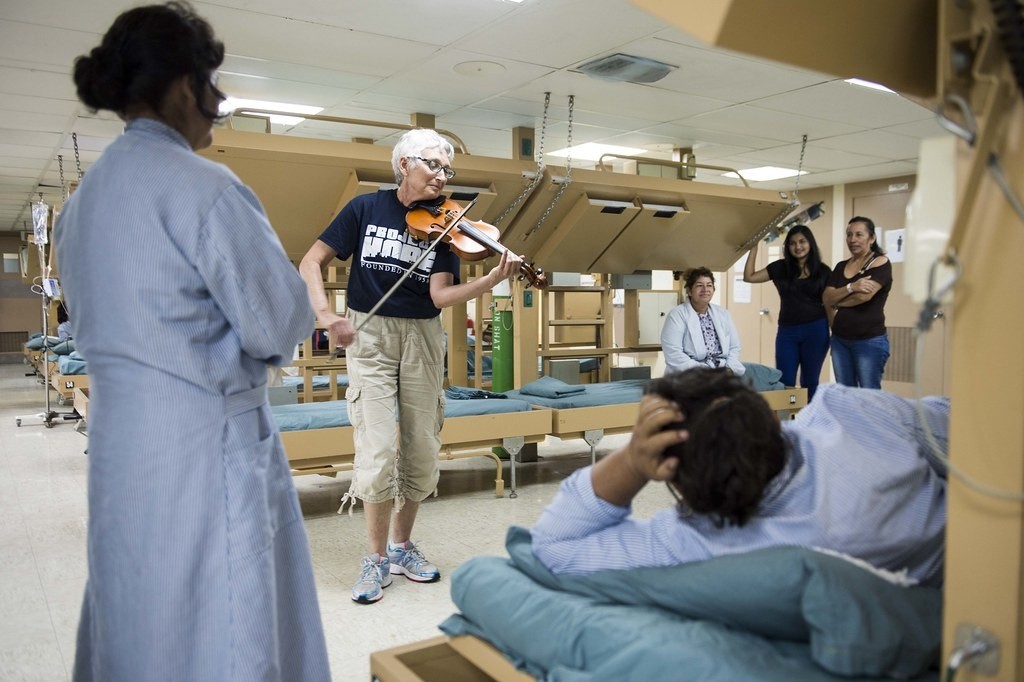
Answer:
[22,321,1024,682]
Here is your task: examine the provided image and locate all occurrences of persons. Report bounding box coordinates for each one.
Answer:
[744,226,832,405]
[533,367,951,589]
[53,0,333,682]
[299,128,526,603]
[823,217,895,392]
[661,266,746,375]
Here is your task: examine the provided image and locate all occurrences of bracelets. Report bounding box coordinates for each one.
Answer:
[847,283,853,294]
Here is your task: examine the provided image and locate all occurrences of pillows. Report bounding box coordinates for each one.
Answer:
[26,337,54,348]
[52,340,74,355]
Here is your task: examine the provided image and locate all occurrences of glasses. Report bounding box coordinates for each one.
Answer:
[406,156,456,179]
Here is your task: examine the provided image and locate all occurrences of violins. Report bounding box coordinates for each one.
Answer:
[405,194,550,292]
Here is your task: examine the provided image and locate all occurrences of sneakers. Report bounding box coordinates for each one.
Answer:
[352,553,393,604]
[386,540,441,582]
